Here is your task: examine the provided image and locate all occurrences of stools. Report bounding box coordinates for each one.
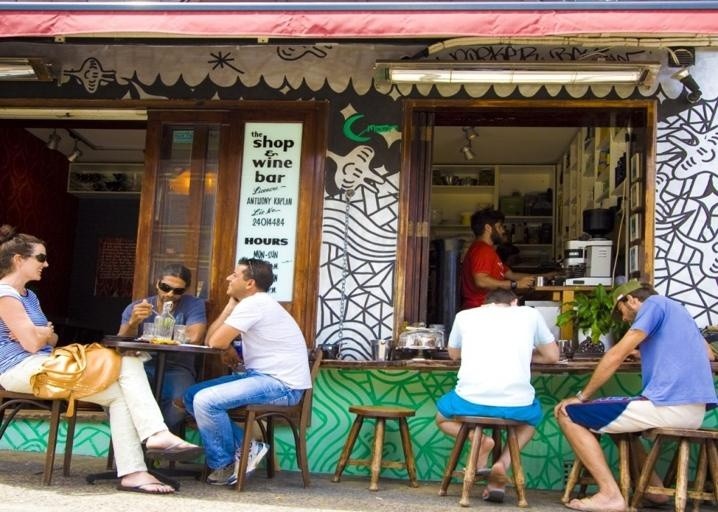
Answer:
[631,429,717,512]
[334,405,418,490]
[438,416,527,506]
[563,429,641,509]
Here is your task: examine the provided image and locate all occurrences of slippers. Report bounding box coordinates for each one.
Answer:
[146,439,203,464]
[462,467,492,477]
[565,497,627,511]
[482,486,505,503]
[117,482,175,495]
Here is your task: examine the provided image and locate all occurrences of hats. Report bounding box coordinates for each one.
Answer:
[610,278,650,321]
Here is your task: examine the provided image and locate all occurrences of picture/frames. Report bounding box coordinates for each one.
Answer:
[629,153,640,275]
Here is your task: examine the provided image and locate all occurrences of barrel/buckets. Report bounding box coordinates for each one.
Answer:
[370,339,396,361]
[582,208,615,238]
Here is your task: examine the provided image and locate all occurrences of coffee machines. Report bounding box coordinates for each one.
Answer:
[557,209,612,286]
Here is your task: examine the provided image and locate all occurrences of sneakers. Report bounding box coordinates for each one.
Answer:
[206,463,234,486]
[227,437,270,489]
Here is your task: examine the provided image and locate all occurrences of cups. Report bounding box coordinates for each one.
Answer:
[142,322,155,339]
[371,339,394,362]
[536,276,544,287]
[557,340,568,353]
[460,211,473,225]
[173,323,187,343]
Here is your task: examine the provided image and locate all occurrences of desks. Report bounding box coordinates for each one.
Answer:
[529,284,616,353]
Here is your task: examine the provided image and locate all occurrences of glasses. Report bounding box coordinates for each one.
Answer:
[20,251,48,264]
[247,256,255,278]
[157,280,186,296]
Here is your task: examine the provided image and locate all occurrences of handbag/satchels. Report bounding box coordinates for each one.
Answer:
[29,340,122,417]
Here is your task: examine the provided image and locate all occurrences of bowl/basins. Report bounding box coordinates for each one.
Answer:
[318,343,339,359]
[440,175,459,185]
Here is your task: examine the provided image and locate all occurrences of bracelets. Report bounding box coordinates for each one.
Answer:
[576,390,589,401]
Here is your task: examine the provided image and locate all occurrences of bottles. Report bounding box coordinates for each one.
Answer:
[153,301,175,340]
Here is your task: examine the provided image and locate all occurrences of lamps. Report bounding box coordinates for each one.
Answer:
[461,140,477,161]
[670,67,702,104]
[377,63,660,88]
[46,129,83,164]
[0,55,52,81]
[462,125,477,139]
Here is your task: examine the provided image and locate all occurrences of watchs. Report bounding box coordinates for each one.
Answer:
[511,280,516,290]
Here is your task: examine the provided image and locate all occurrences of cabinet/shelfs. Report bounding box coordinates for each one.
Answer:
[67,162,146,198]
[556,127,629,269]
[430,165,497,242]
[498,164,555,275]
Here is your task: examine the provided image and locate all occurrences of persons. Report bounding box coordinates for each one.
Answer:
[0,225,205,494]
[118,264,207,428]
[182,257,313,486]
[461,209,557,309]
[434,286,561,503]
[554,277,718,512]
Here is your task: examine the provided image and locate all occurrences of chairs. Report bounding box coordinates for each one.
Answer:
[0,382,77,483]
[231,350,323,490]
